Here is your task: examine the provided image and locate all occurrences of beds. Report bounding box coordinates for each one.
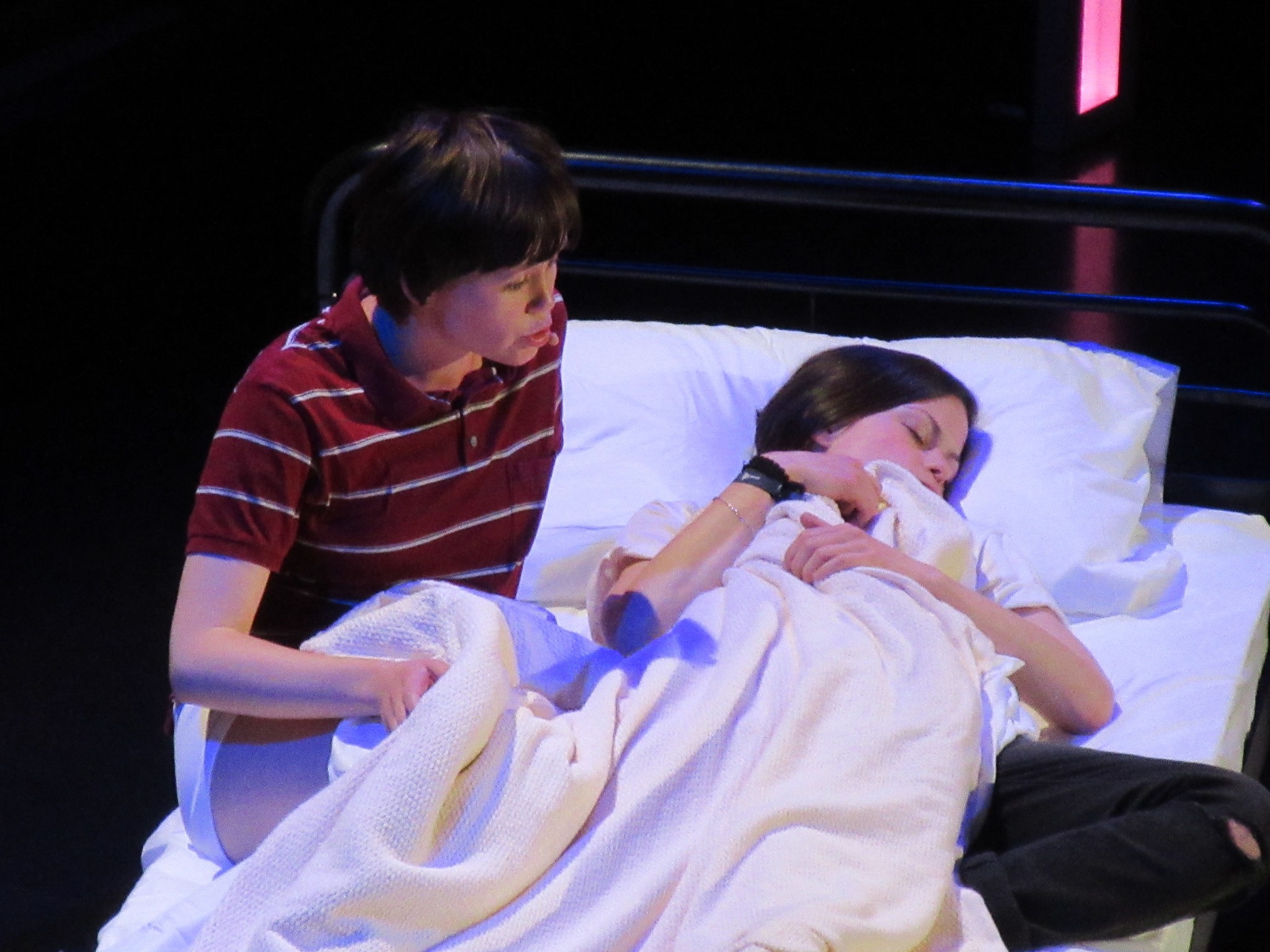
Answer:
[95,138,1267,952]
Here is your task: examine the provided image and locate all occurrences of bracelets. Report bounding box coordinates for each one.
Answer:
[732,452,808,501]
[708,494,757,544]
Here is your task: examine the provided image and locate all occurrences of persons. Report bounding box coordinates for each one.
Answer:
[580,346,1269,950]
[165,105,571,868]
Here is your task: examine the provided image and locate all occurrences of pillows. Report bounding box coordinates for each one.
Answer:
[519,296,1189,648]
